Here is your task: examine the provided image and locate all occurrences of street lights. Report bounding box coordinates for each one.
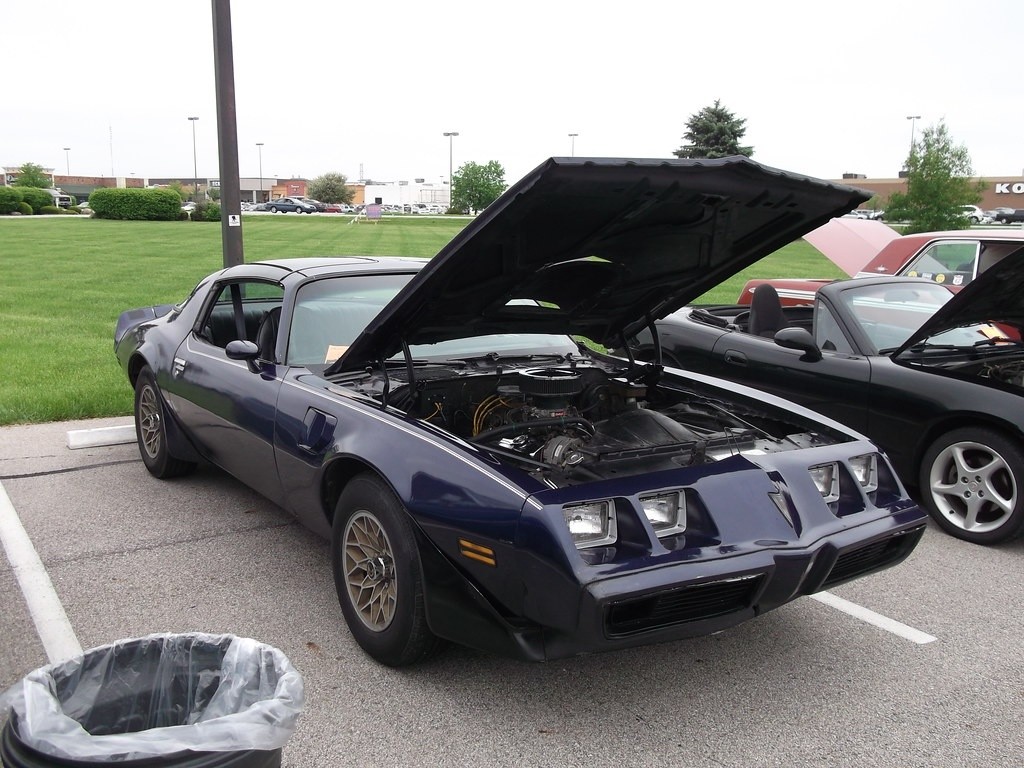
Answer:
[65,147,70,176]
[569,134,578,157]
[256,143,264,202]
[188,117,199,200]
[444,132,459,209]
[907,116,921,177]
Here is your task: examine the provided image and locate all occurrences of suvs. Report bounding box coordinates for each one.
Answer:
[953,205,985,224]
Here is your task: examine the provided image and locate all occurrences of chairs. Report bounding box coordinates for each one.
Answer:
[748,284,792,340]
[253,305,332,364]
[822,278,854,354]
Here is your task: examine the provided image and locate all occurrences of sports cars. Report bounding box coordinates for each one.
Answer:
[114,154,931,662]
[622,244,1024,548]
[736,216,1023,344]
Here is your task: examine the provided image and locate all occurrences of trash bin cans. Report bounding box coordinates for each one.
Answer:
[0,629,292,768]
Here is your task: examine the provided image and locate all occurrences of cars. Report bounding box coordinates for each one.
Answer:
[266,197,317,214]
[850,208,888,222]
[240,201,270,212]
[985,206,1024,224]
[180,202,197,211]
[290,195,449,215]
[42,189,72,208]
